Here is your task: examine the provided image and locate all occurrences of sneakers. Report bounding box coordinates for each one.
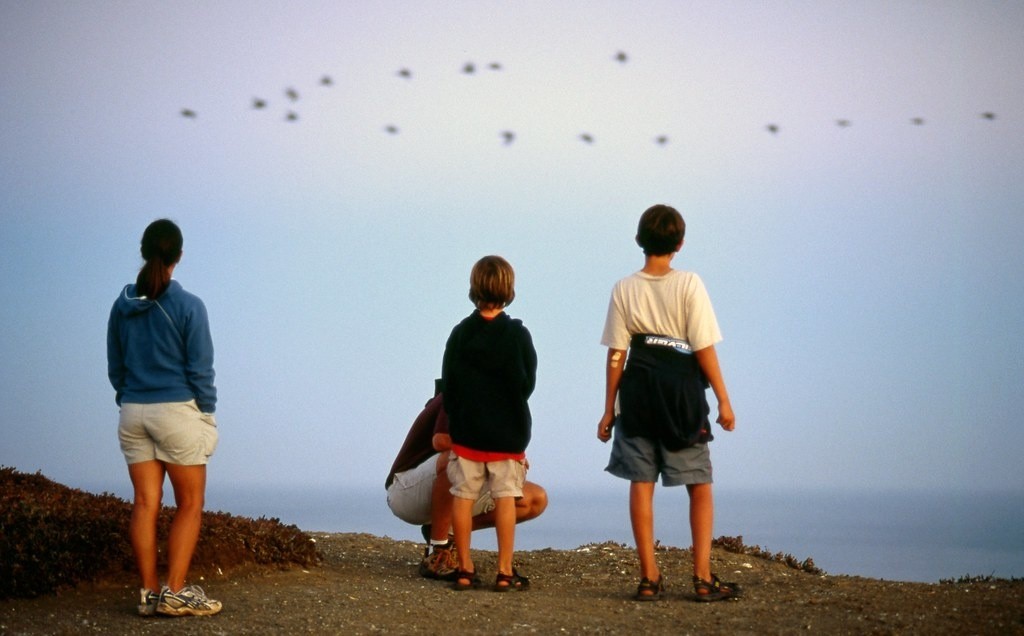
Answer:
[419,544,459,579]
[158,583,222,616]
[137,588,160,615]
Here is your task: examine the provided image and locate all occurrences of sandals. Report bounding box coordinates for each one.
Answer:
[456,567,482,590]
[638,575,666,601]
[495,568,528,592]
[692,574,740,602]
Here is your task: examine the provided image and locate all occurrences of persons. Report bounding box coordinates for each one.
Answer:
[597,203,741,604]
[382,374,547,581]
[107,217,222,615]
[441,255,539,592]
